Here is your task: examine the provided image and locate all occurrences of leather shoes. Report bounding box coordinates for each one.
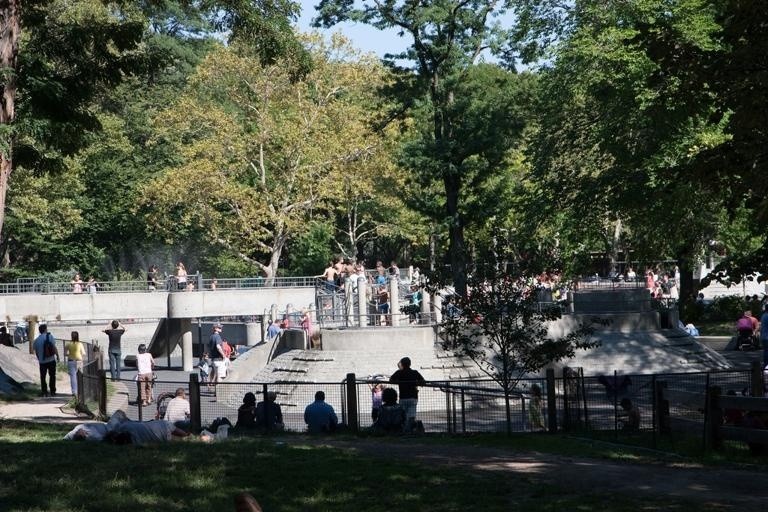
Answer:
[42,392,57,397]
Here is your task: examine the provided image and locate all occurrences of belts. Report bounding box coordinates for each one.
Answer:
[67,359,83,362]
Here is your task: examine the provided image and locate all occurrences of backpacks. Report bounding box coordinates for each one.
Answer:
[42,332,55,361]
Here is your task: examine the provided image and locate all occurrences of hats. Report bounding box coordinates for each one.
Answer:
[213,322,224,328]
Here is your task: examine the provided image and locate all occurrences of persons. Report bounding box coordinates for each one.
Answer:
[234,492,264,511]
